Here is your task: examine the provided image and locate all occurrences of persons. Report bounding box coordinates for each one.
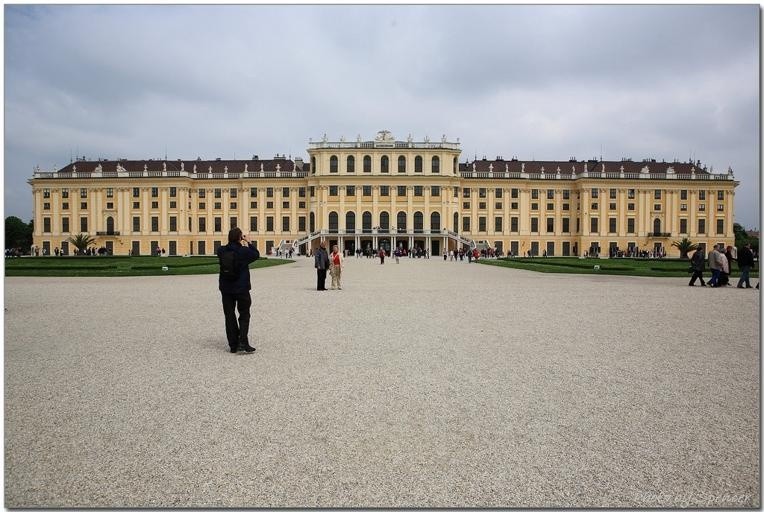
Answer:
[5,246,98,257]
[275,246,294,259]
[354,242,430,264]
[584,246,663,258]
[440,245,548,264]
[328,245,342,290]
[217,227,259,355]
[685,240,754,288]
[314,240,330,292]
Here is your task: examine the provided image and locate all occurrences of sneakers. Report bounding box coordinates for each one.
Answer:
[230,345,256,353]
